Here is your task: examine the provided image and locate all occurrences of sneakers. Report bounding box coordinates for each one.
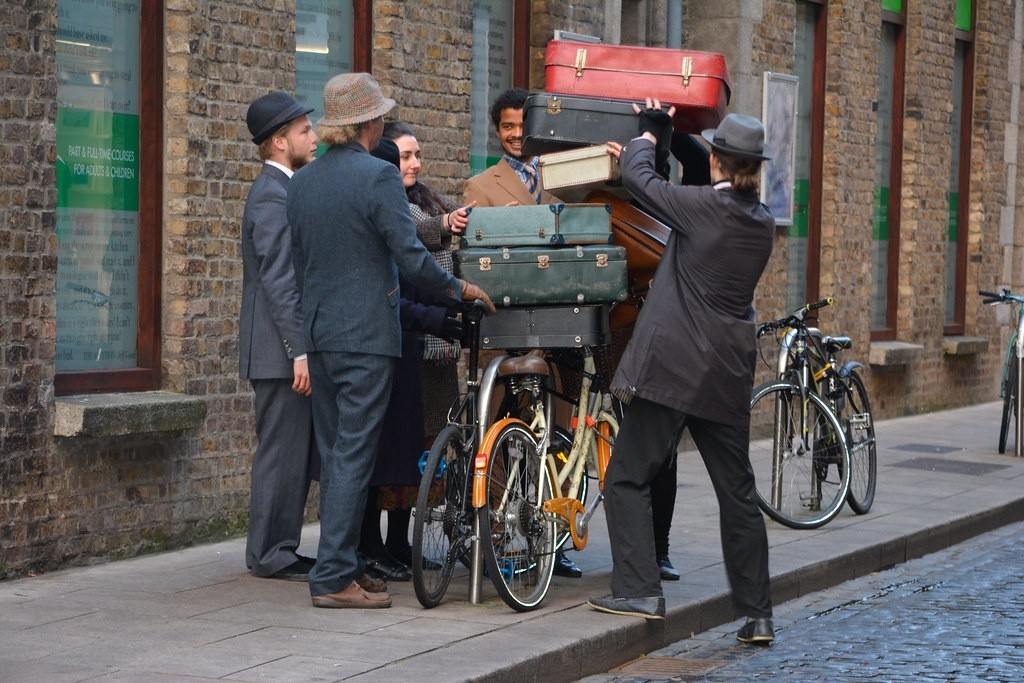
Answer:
[656,559,679,579]
[553,547,582,577]
[312,579,392,609]
[353,573,387,592]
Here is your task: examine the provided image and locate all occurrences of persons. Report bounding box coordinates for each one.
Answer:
[464,89,582,577]
[239,92,320,581]
[358,121,518,580]
[286,72,497,608]
[589,97,778,642]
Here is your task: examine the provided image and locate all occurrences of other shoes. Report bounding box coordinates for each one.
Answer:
[359,554,411,581]
[265,551,317,582]
[364,545,408,571]
[587,594,666,620]
[737,616,774,642]
[384,541,442,569]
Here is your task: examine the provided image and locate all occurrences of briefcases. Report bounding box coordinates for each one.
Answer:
[450,244,628,308]
[537,144,633,201]
[543,39,731,132]
[581,189,673,295]
[460,203,612,243]
[522,93,674,156]
[477,305,611,348]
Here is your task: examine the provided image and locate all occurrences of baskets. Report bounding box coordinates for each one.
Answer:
[552,320,636,397]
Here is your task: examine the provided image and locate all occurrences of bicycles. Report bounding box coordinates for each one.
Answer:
[406,296,629,616]
[977,287,1024,458]
[748,297,878,532]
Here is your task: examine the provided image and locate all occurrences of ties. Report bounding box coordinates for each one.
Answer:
[523,163,540,204]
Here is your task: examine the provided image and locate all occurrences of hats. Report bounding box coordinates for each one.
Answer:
[317,73,395,126]
[701,113,773,160]
[371,137,401,170]
[247,94,315,146]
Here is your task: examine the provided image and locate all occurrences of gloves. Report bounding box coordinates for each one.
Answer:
[437,308,467,345]
